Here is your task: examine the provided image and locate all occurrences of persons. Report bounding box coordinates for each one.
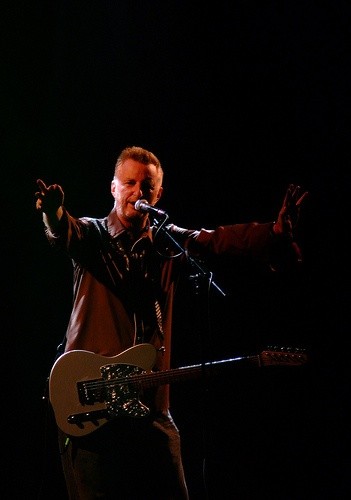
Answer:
[36,146,308,499]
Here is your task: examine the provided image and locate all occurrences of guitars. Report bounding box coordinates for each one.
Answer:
[49,343,311,435]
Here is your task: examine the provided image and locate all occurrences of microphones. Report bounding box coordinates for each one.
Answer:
[135,199,167,216]
